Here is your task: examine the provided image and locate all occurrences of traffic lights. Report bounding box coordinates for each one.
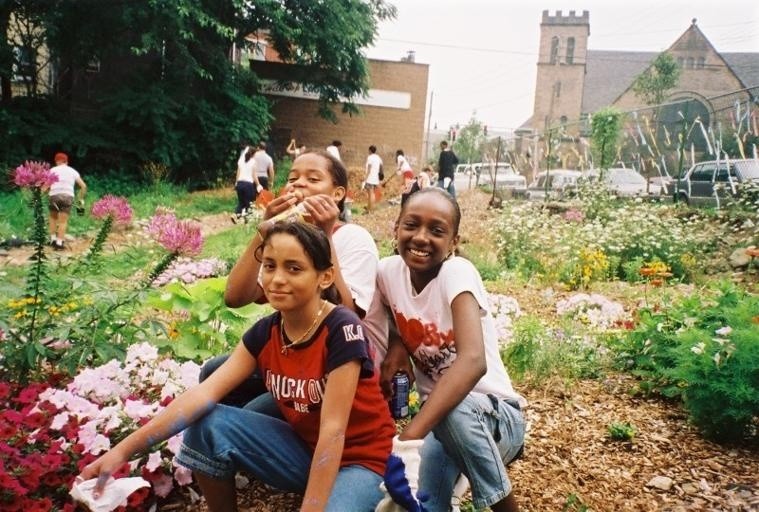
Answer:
[484,125,488,136]
[451,128,456,142]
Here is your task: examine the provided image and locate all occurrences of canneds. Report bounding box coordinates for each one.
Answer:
[387,371,410,419]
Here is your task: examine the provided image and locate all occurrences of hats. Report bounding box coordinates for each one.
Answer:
[54,152,68,163]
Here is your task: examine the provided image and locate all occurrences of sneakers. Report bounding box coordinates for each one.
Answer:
[54,241,67,251]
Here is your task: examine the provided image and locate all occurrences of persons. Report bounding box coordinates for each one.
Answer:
[42,153,87,251]
[72,224,399,512]
[360,186,529,512]
[198,147,380,410]
[228,137,459,225]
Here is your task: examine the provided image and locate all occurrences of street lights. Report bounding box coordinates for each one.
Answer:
[425,88,438,160]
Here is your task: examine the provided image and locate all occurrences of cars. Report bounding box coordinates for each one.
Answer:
[456,162,672,199]
[669,159,759,207]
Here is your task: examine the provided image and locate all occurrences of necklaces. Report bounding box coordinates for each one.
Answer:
[279,300,328,356]
